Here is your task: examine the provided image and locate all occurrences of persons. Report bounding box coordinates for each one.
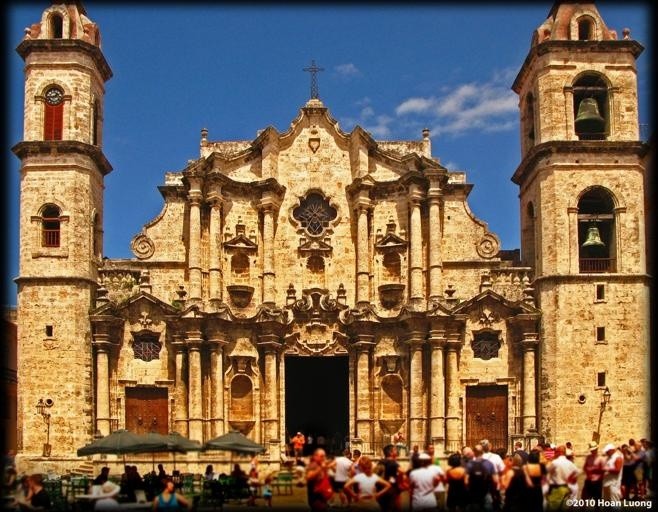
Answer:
[0,455,656,512]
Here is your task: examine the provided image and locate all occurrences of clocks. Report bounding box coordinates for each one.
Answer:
[45,88,64,106]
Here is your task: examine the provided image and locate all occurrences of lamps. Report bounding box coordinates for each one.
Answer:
[594,387,610,401]
[35,398,51,418]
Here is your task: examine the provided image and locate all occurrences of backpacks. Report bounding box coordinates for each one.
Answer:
[469,460,487,496]
[372,458,395,485]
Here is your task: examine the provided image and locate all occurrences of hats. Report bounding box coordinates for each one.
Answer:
[587,441,616,454]
[481,437,491,448]
[417,452,432,461]
[98,481,121,497]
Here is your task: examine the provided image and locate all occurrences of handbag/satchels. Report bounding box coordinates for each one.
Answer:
[263,490,272,497]
[313,472,336,502]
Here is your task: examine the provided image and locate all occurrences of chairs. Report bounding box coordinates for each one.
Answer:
[41,471,295,507]
